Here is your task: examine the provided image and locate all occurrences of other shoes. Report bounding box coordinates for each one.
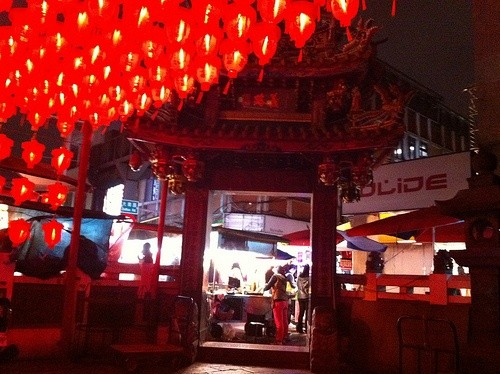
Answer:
[270,339,285,345]
[296,329,304,333]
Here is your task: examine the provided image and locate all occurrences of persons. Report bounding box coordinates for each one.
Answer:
[204,258,221,291]
[137,242,154,264]
[167,257,180,282]
[228,261,247,295]
[263,264,297,346]
[296,264,311,334]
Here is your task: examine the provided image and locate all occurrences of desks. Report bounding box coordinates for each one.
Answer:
[212,294,296,338]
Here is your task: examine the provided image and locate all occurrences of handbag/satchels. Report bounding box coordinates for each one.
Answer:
[271,299,275,308]
